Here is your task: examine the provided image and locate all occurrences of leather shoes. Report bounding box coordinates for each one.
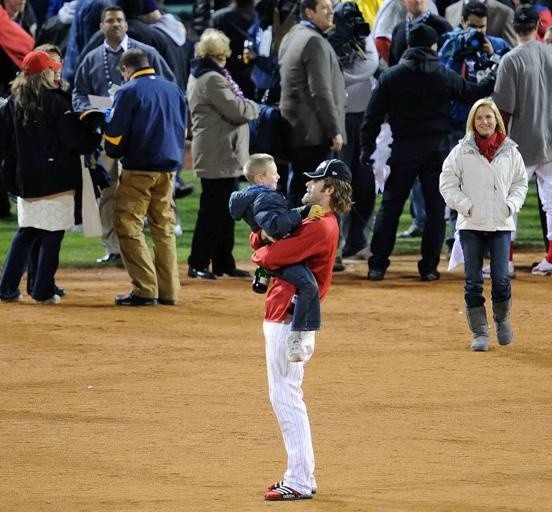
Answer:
[54,288,65,296]
[217,268,249,277]
[158,297,177,305]
[115,291,157,306]
[188,266,217,279]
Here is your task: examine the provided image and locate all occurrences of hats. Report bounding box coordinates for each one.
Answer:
[21,50,61,75]
[514,4,544,26]
[407,23,439,47]
[304,158,352,184]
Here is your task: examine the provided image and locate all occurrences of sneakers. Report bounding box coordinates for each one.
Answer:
[265,486,313,500]
[174,224,183,236]
[36,294,60,304]
[174,185,193,198]
[332,228,440,281]
[532,258,552,276]
[267,480,316,494]
[483,260,516,277]
[285,331,305,362]
[97,253,121,265]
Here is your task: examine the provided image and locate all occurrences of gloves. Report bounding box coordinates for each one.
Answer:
[306,202,325,221]
[360,147,376,169]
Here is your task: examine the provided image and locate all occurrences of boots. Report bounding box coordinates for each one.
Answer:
[493,297,512,345]
[466,306,491,350]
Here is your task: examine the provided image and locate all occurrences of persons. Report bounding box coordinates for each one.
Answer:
[440,99,529,353]
[228,154,322,361]
[249,159,355,499]
[2,1,552,306]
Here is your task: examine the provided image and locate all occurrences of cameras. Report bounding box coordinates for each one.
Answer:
[455,28,486,69]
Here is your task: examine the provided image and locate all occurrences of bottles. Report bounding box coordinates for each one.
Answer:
[252,263,272,294]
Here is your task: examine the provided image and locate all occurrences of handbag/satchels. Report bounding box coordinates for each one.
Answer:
[249,103,281,155]
